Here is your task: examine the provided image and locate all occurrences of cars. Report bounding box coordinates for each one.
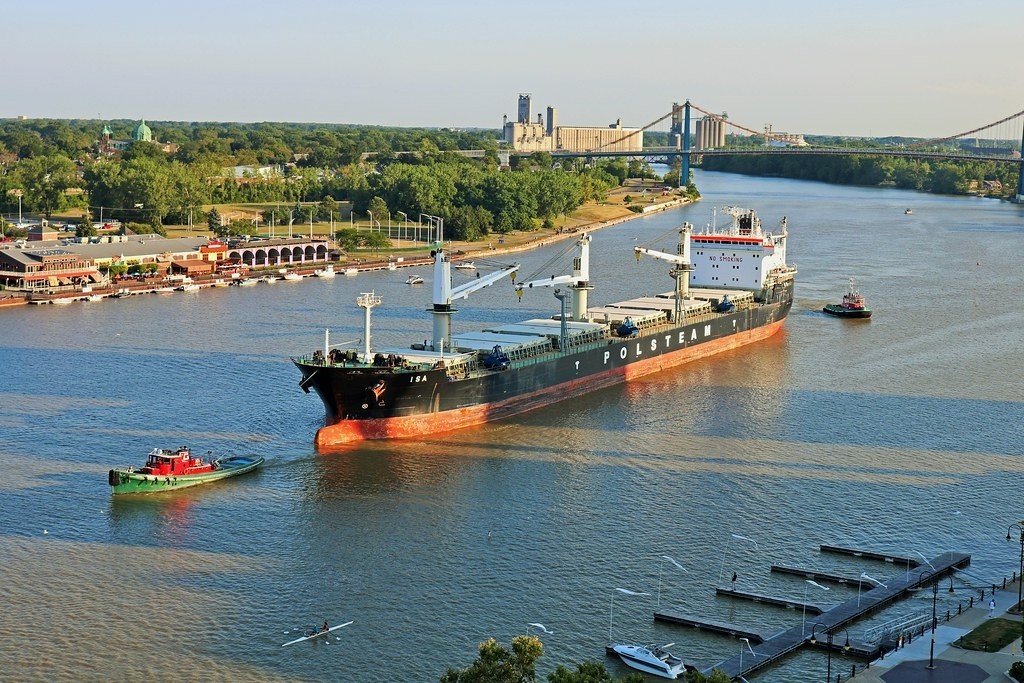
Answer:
[133,200,144,210]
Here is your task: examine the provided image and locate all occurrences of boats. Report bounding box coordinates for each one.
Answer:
[109,445,265,498]
[239,278,259,288]
[288,204,799,449]
[114,290,134,300]
[823,277,873,319]
[50,296,75,305]
[85,294,103,303]
[285,270,308,281]
[607,640,688,681]
[904,207,913,215]
[454,260,477,271]
[316,269,335,278]
[405,275,424,285]
[261,274,277,285]
[343,267,359,276]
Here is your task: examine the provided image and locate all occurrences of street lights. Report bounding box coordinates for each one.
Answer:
[811,622,851,683]
[1005,523,1024,612]
[917,570,955,667]
